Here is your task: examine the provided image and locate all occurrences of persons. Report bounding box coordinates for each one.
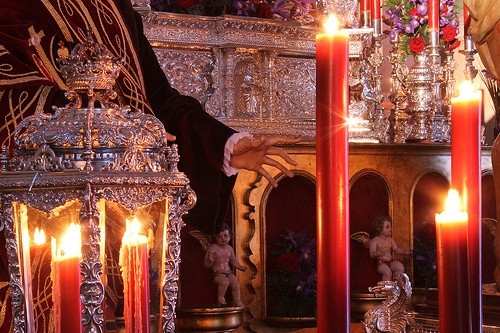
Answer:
[0,0,301,235]
[370,215,413,283]
[203,221,247,307]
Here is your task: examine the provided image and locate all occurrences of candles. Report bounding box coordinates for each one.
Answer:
[360,0,370,11]
[316,13,350,333]
[50,223,82,333]
[427,0,440,29]
[370,0,380,19]
[119,217,149,333]
[450,81,482,333]
[435,188,473,333]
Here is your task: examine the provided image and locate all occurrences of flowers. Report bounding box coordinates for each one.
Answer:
[380,0,461,63]
[269,228,316,313]
